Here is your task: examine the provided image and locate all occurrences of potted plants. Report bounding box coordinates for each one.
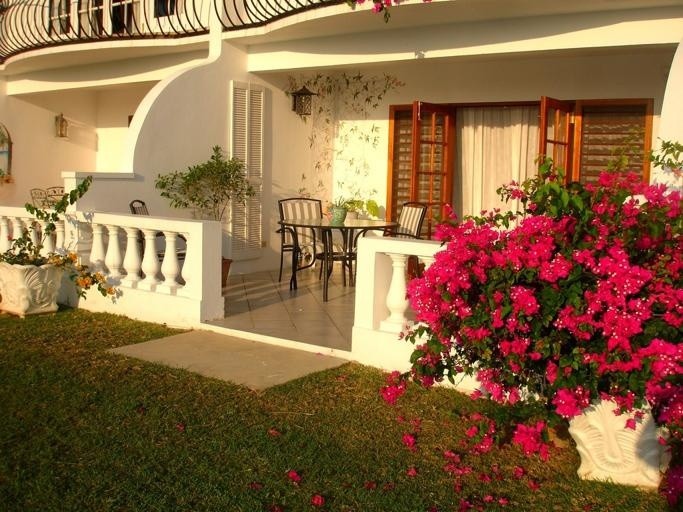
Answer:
[326,196,381,224]
[155,145,255,287]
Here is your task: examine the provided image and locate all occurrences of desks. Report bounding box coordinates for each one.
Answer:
[277,218,401,302]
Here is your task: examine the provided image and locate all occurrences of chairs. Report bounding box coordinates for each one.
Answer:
[353,202,427,287]
[130,199,186,277]
[276,198,325,282]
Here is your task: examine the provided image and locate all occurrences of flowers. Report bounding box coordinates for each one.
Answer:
[0,176,117,300]
[380,155,683,509]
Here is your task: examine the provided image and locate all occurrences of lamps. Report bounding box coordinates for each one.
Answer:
[290,84,319,115]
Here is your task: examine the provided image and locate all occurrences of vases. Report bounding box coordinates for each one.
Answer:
[0,261,62,319]
[568,395,672,493]
